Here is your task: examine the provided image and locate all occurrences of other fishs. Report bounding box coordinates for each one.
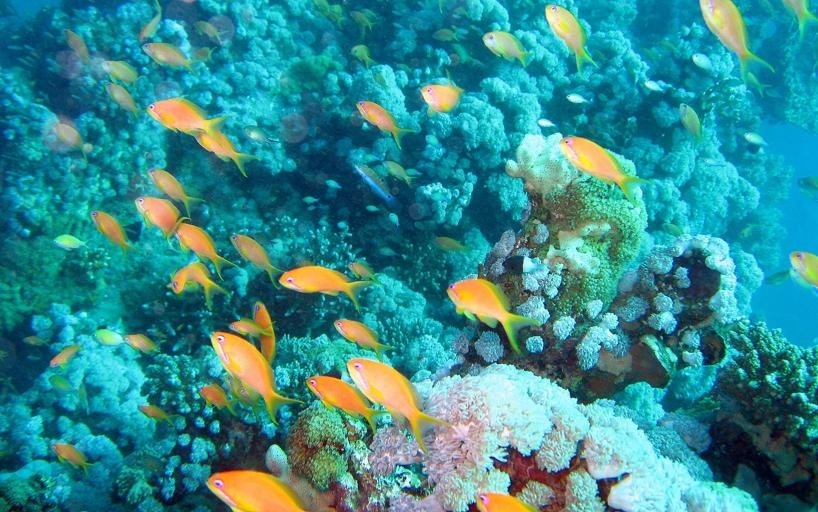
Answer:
[7,0,818,512]
